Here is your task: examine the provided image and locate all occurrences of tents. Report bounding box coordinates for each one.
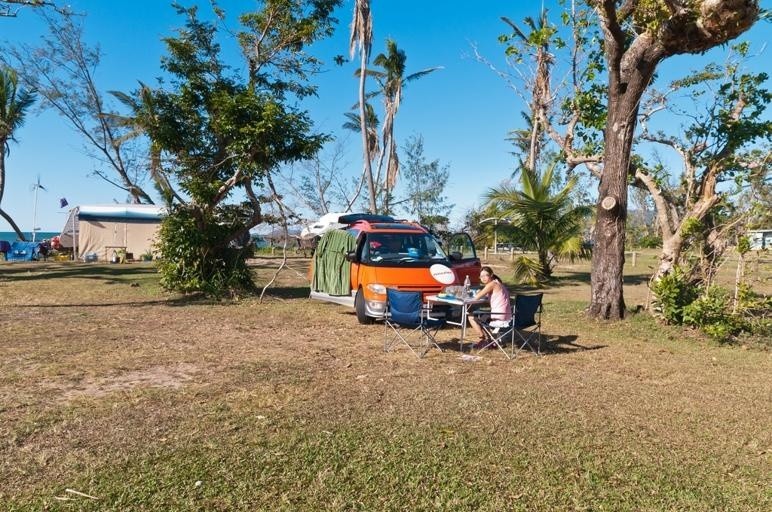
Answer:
[4,241,47,262]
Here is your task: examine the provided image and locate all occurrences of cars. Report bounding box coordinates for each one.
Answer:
[307,213,482,324]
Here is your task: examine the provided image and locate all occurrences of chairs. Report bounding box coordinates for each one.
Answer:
[472,292,544,361]
[384,287,445,360]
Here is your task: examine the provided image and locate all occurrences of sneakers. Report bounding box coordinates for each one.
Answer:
[477,339,497,349]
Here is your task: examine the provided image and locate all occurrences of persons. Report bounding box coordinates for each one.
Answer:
[467,267,513,349]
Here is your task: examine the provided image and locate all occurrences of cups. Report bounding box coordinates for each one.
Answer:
[466,291,473,299]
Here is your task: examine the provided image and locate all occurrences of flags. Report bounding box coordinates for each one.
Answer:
[60,198,68,208]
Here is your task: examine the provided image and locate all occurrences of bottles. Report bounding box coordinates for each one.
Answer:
[464,275,471,289]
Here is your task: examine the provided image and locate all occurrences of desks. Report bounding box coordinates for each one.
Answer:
[425,289,488,352]
